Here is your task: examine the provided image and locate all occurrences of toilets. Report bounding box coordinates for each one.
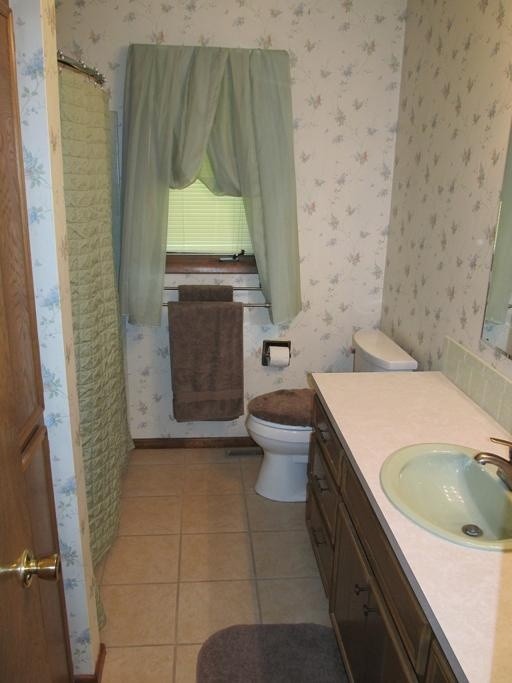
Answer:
[246,326,417,502]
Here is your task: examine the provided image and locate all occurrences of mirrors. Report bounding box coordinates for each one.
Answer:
[480,119,512,359]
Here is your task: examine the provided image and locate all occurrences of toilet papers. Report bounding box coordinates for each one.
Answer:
[265,345,289,369]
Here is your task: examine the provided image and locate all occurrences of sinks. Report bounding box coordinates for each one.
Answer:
[380,442,512,552]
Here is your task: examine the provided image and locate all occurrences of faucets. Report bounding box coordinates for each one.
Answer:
[474,437,511,490]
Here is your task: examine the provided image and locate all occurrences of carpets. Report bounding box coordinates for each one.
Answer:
[196,620,349,682]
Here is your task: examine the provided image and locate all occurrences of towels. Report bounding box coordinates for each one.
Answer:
[167,282,246,423]
